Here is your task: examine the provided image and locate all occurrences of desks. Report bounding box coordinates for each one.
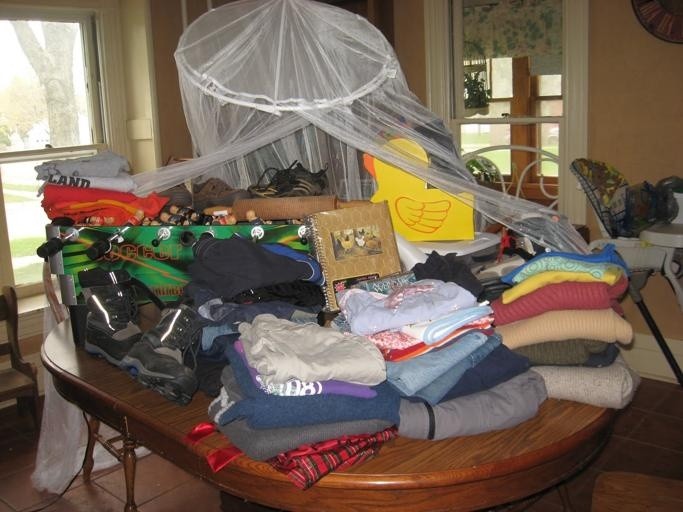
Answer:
[40,301,616,512]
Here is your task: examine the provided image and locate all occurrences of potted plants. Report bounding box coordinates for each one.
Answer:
[465,65,489,118]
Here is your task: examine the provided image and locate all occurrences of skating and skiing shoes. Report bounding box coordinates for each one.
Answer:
[77,268,202,407]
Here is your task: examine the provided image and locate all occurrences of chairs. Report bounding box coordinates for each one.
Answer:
[569,148,681,386]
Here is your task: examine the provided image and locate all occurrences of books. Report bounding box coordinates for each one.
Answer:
[304,200,404,313]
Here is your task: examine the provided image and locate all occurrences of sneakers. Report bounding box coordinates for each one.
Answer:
[158,162,329,211]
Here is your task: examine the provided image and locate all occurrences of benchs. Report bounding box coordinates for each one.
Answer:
[0,287,42,439]
